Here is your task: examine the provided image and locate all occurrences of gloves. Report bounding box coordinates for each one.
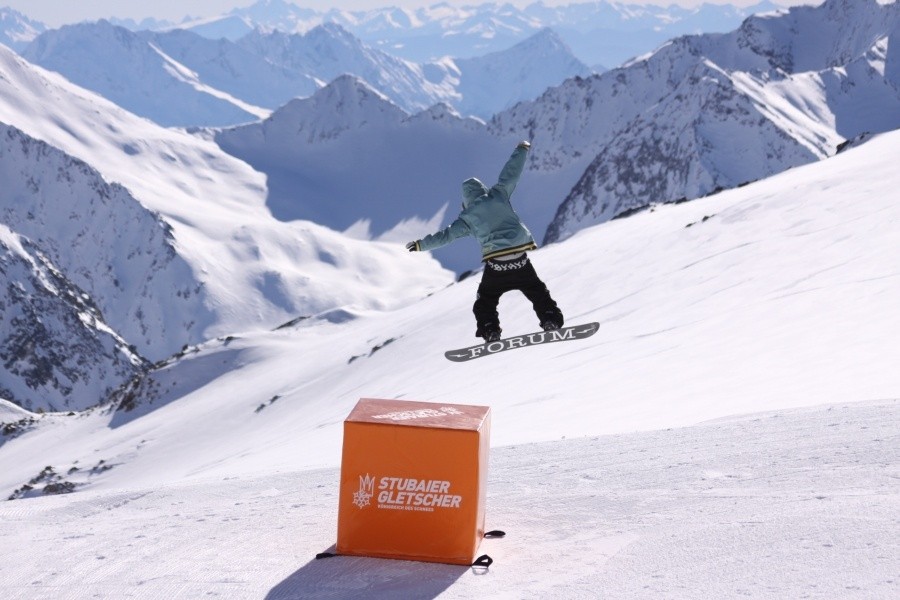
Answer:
[518,141,531,150]
[406,241,421,251]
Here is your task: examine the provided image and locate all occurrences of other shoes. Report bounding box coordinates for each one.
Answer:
[543,321,560,331]
[485,328,502,343]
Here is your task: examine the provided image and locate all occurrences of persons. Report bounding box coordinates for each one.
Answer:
[404,140,564,344]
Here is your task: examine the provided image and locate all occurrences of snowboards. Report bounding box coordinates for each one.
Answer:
[443,322,601,363]
